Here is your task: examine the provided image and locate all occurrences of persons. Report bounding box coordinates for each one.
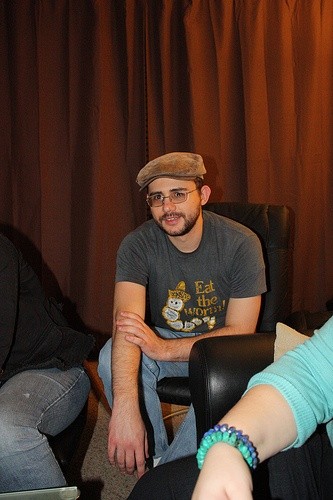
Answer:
[98,152,267,480]
[0,214,112,495]
[191,314,333,500]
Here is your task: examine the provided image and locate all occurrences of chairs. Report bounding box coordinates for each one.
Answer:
[157,203,296,406]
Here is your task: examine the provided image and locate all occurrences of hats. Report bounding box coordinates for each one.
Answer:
[137,152,206,191]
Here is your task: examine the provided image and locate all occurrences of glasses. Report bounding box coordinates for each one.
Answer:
[146,188,199,208]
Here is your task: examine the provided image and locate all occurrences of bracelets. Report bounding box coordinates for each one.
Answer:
[197,425,259,470]
